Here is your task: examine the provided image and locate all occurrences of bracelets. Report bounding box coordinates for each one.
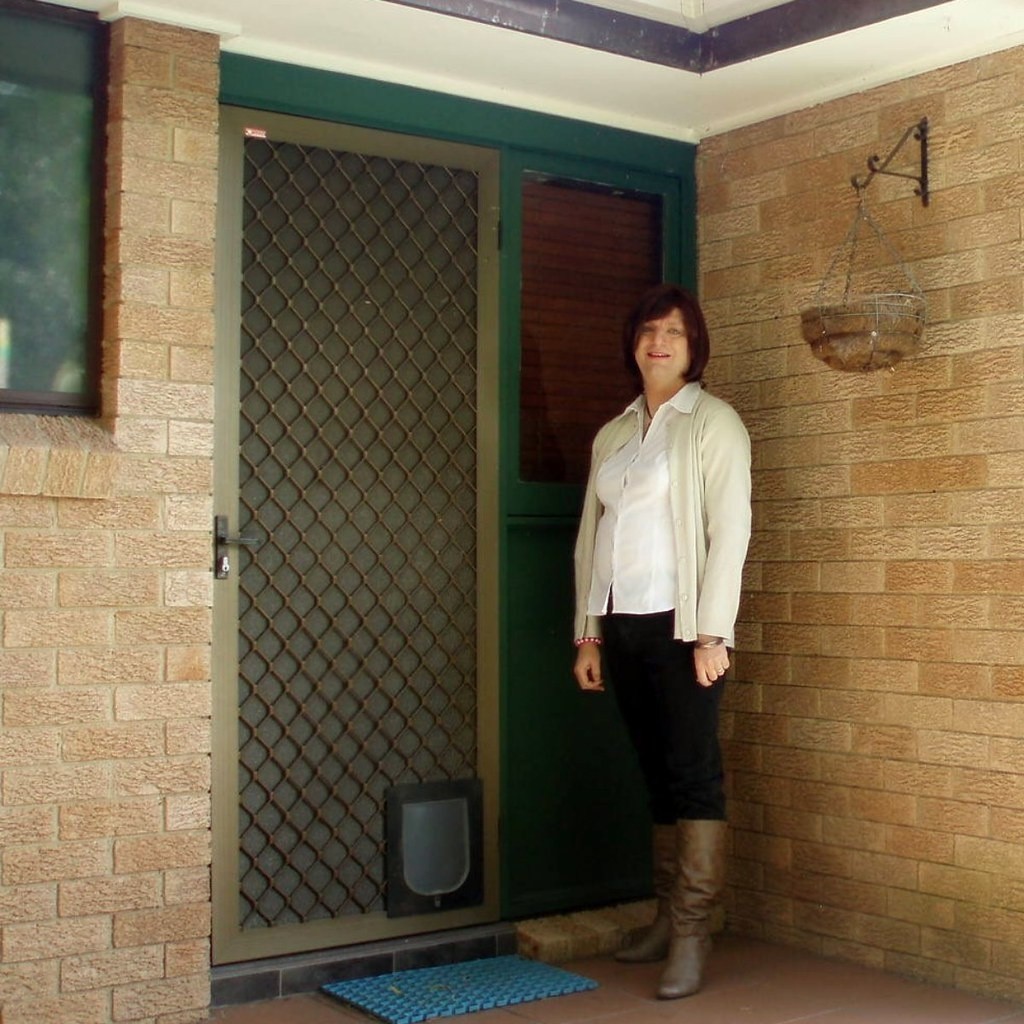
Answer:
[575,638,603,648]
[694,636,722,649]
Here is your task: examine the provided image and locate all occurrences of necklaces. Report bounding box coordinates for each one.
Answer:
[645,399,655,420]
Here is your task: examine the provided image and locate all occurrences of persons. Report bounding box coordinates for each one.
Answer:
[570,288,754,998]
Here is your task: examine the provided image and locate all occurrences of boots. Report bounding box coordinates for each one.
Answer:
[655,819,731,999]
[615,816,683,964]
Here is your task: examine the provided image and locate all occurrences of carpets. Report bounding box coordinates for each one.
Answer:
[320,953,599,1024]
